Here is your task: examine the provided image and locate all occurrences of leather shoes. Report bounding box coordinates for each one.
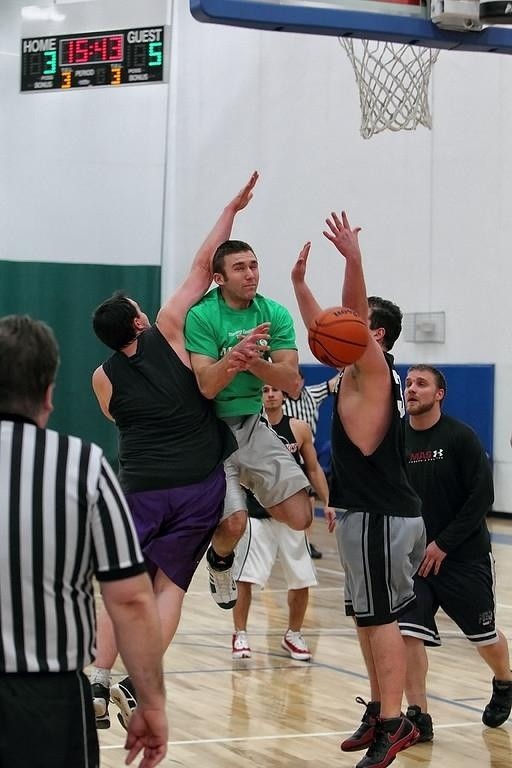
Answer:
[310,544,321,558]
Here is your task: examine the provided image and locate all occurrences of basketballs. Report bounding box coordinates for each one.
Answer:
[309,308,368,365]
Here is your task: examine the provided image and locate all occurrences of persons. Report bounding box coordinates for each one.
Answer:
[183,240,313,609]
[291,212,420,768]
[83,170,257,729]
[232,384,338,661]
[258,368,342,559]
[399,363,512,743]
[1,313,168,768]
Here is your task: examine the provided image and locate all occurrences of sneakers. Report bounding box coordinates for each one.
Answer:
[341,702,433,768]
[206,545,239,609]
[91,676,137,732]
[482,670,512,728]
[232,631,252,658]
[280,629,312,660]
